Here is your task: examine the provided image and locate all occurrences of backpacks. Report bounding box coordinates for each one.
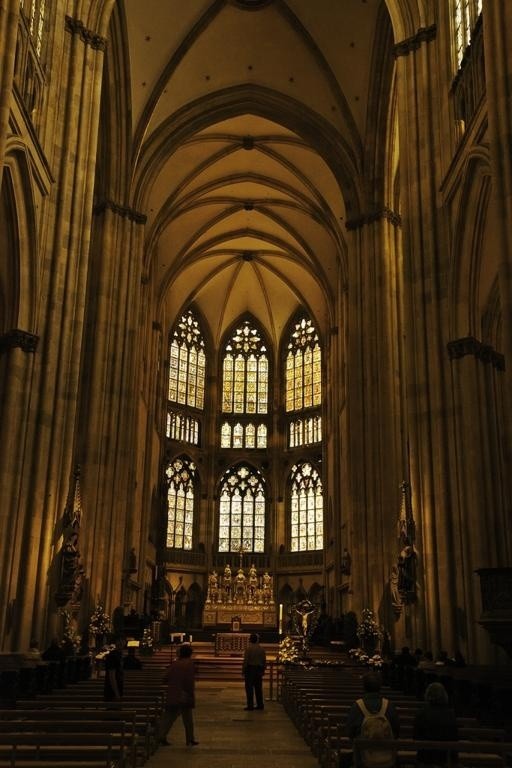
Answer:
[356,698,395,768]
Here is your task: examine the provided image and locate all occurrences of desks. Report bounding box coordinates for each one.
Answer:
[215,633,260,657]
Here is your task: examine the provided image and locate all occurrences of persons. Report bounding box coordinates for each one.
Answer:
[348,670,401,767]
[122,646,143,671]
[295,608,318,635]
[41,637,65,689]
[25,638,40,651]
[157,644,200,745]
[392,646,460,699]
[101,630,126,715]
[240,632,267,710]
[414,681,459,768]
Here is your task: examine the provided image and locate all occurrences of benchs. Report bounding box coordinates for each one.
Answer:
[276,666,512,768]
[0,650,172,768]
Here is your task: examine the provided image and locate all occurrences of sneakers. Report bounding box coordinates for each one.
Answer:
[159,740,199,746]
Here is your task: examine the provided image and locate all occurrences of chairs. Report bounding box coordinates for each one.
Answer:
[229,616,243,633]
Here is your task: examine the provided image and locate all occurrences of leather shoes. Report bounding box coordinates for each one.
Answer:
[243,707,264,711]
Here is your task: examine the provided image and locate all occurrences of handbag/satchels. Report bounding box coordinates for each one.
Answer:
[180,690,193,709]
